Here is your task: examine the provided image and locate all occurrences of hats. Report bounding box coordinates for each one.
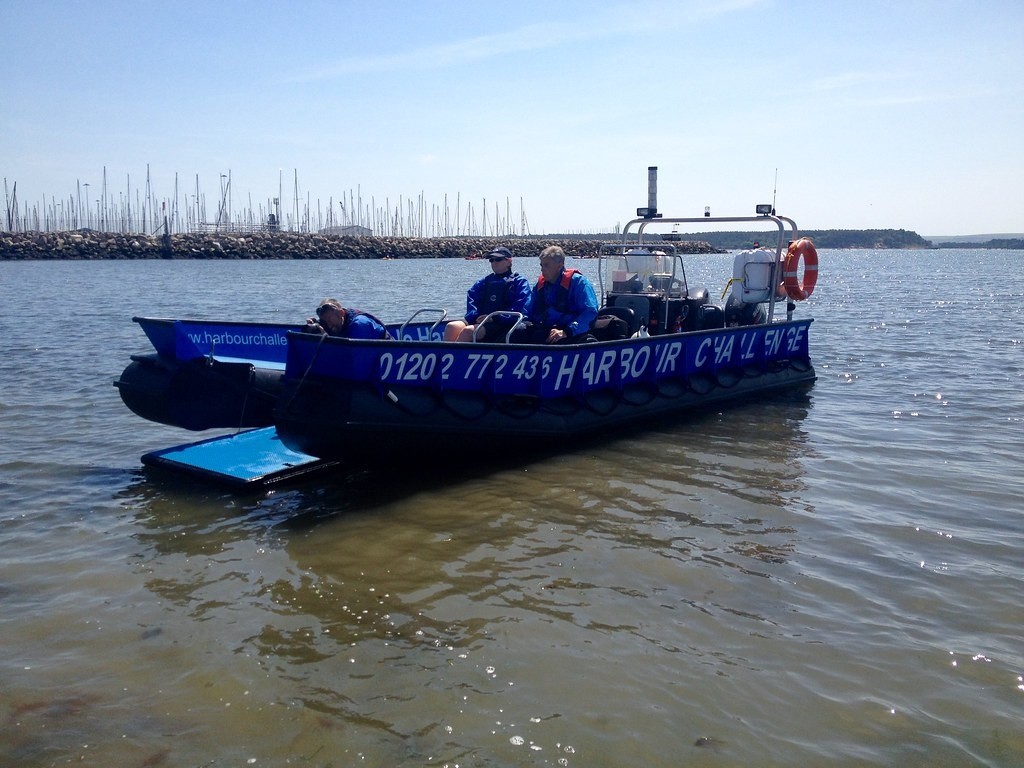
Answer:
[486,247,512,259]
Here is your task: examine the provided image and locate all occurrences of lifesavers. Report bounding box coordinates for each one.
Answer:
[783,237,819,302]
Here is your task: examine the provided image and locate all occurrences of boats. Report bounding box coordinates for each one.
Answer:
[109,168,821,496]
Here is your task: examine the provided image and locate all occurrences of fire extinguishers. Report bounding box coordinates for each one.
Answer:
[672,316,681,333]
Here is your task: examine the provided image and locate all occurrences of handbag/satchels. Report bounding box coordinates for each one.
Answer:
[592,314,628,341]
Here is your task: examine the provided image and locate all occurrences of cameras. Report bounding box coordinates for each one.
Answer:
[308,319,327,334]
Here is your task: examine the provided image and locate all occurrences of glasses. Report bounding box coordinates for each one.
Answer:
[489,257,505,263]
[316,304,333,316]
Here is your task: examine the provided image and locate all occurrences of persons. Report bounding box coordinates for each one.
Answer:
[521,244,599,345]
[305,298,396,340]
[442,247,532,343]
